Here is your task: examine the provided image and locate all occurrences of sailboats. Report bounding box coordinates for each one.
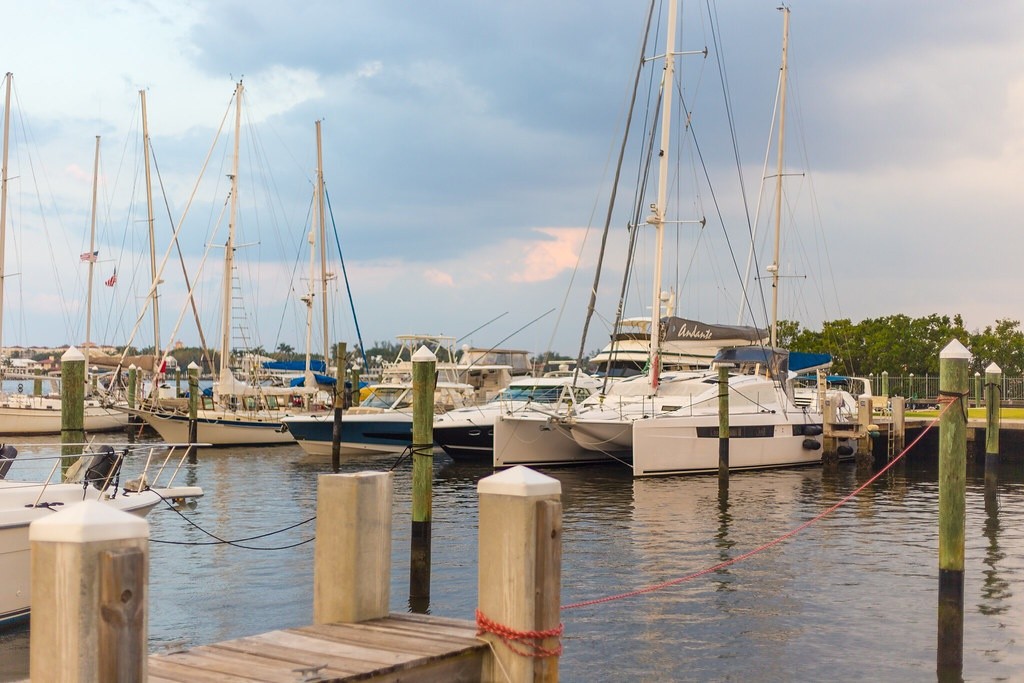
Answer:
[1,0,887,475]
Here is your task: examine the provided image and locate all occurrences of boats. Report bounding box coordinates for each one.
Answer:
[0,441,212,618]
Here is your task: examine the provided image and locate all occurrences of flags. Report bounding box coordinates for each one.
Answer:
[105,266,117,286]
[81,251,98,262]
